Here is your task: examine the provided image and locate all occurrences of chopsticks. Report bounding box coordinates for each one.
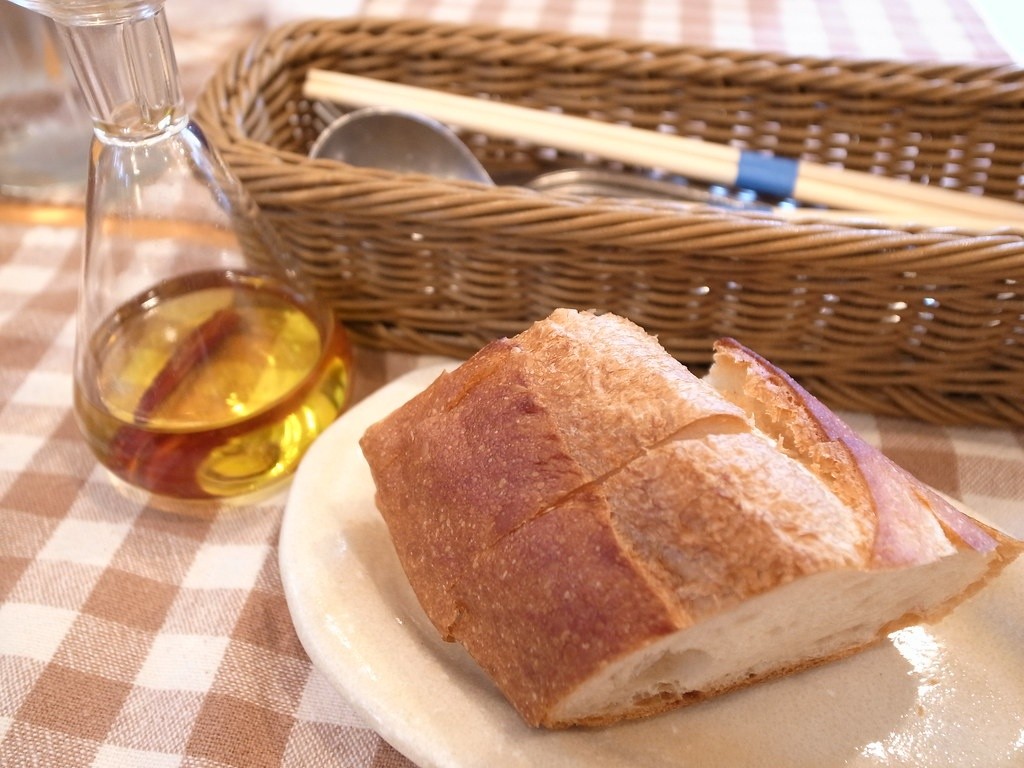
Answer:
[303,63,1024,234]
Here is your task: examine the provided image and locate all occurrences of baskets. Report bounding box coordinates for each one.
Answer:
[186,17,1024,431]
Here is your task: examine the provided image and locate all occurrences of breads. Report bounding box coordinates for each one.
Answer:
[357,304,1024,730]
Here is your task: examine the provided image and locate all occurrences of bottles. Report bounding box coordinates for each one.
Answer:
[3,0,355,511]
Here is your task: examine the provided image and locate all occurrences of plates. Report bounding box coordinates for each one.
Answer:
[275,360,1023,768]
